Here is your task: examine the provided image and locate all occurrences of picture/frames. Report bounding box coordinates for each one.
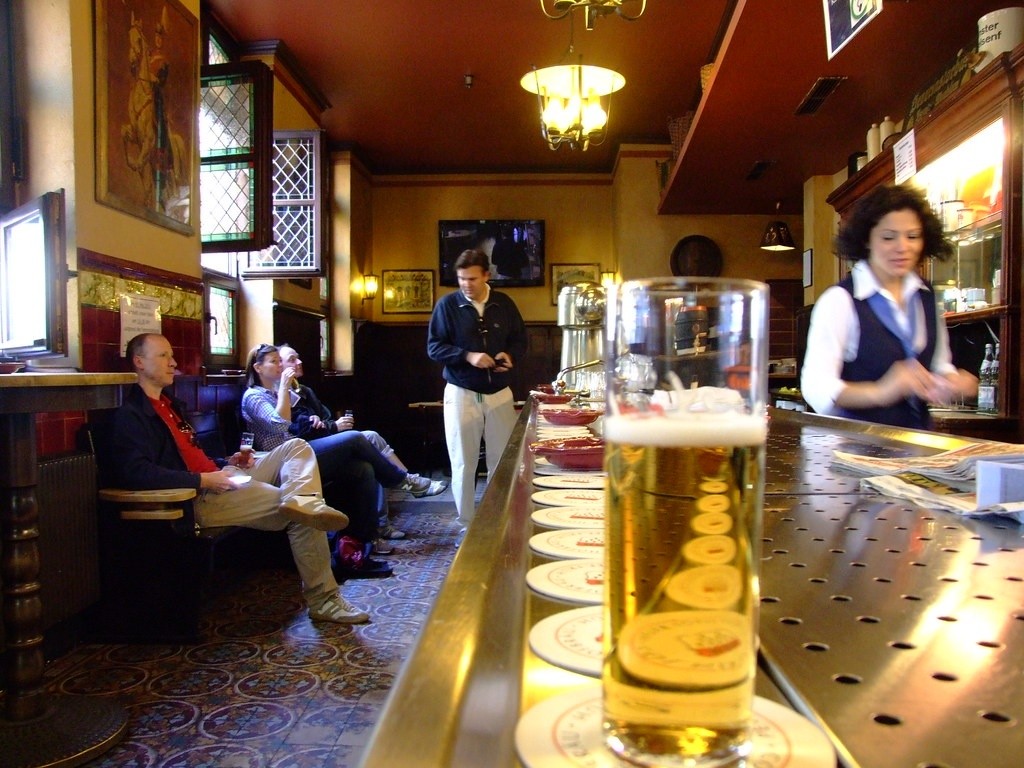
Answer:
[382,269,436,314]
[94,0,199,237]
[550,263,602,305]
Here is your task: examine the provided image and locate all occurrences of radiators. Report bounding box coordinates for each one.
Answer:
[35,454,101,631]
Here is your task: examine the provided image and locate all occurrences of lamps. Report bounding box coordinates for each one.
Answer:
[361,272,380,305]
[761,220,795,251]
[519,1,648,151]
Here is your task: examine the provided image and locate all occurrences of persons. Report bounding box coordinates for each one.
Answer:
[491,232,524,281]
[800,188,977,441]
[428,249,526,548]
[241,343,449,555]
[98,333,370,625]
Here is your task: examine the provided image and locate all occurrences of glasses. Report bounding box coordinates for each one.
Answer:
[177,420,199,447]
[254,343,268,359]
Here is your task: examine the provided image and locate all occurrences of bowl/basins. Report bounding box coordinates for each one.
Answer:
[531,394,572,404]
[882,132,903,151]
[536,384,554,394]
[537,409,603,425]
[977,6,1024,71]
[529,437,605,471]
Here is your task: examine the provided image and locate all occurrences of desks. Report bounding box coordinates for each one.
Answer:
[410,401,524,478]
[0,369,139,768]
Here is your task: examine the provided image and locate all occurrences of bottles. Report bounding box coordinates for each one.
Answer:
[866,124,880,161]
[880,116,894,152]
[989,342,1000,414]
[978,344,995,415]
[856,156,867,171]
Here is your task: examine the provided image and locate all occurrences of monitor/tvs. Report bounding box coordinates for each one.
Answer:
[438,219,545,286]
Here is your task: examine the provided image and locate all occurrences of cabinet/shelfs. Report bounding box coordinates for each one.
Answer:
[825,40,1024,444]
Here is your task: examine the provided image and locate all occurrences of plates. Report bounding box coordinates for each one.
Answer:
[0,362,26,374]
[514,404,837,768]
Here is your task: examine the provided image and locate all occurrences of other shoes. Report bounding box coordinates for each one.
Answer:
[455,531,466,547]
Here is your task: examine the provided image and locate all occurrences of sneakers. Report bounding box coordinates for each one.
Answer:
[279,495,349,532]
[371,538,394,554]
[413,480,449,498]
[307,592,370,624]
[377,525,405,538]
[392,473,431,493]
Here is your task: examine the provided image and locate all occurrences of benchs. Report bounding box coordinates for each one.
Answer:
[84,405,259,647]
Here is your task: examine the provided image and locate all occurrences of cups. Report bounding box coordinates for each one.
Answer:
[237,432,254,468]
[291,376,300,393]
[345,410,353,417]
[603,277,770,768]
